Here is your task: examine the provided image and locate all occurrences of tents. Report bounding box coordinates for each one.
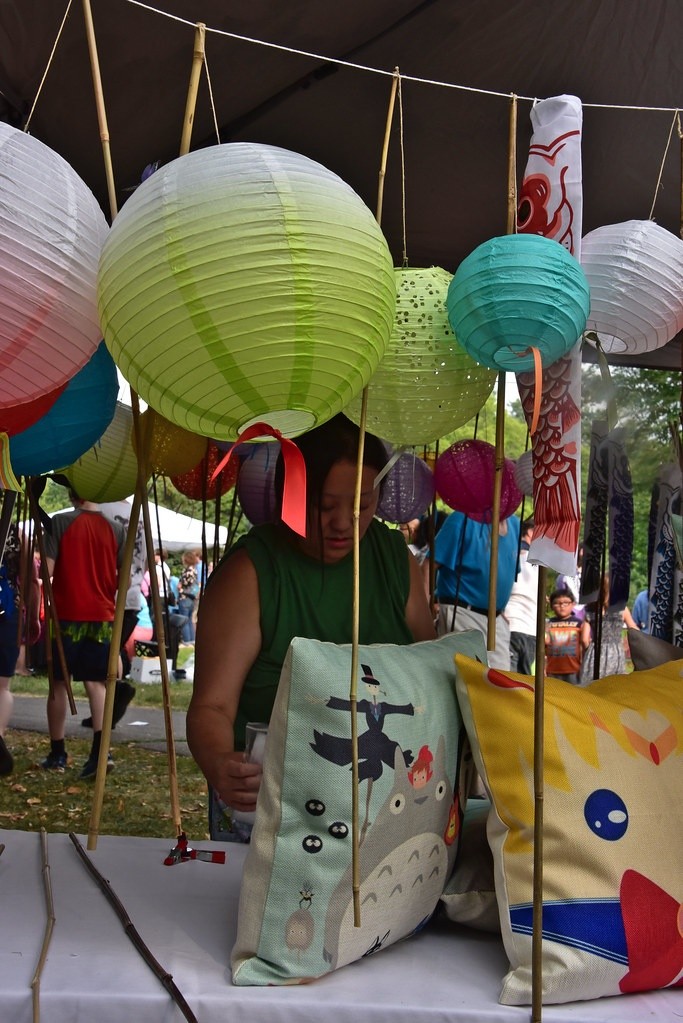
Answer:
[15,492,228,557]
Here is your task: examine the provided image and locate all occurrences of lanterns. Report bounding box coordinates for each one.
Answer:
[0,120,683,523]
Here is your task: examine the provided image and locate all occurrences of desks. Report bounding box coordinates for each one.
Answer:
[0,829,683,1023]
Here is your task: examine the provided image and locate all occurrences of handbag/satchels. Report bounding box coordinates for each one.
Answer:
[168,592,175,606]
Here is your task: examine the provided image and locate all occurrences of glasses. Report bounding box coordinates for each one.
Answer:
[553,601,573,607]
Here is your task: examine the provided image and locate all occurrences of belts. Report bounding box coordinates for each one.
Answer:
[434,596,488,616]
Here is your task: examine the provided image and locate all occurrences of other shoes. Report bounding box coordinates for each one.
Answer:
[81,751,114,783]
[82,717,93,727]
[41,752,70,770]
[0,736,14,773]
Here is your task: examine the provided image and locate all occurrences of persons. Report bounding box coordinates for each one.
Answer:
[187,412,437,844]
[401,510,683,800]
[0,485,207,779]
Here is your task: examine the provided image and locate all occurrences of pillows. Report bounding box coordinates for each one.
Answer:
[453,653,683,1007]
[228,631,490,988]
